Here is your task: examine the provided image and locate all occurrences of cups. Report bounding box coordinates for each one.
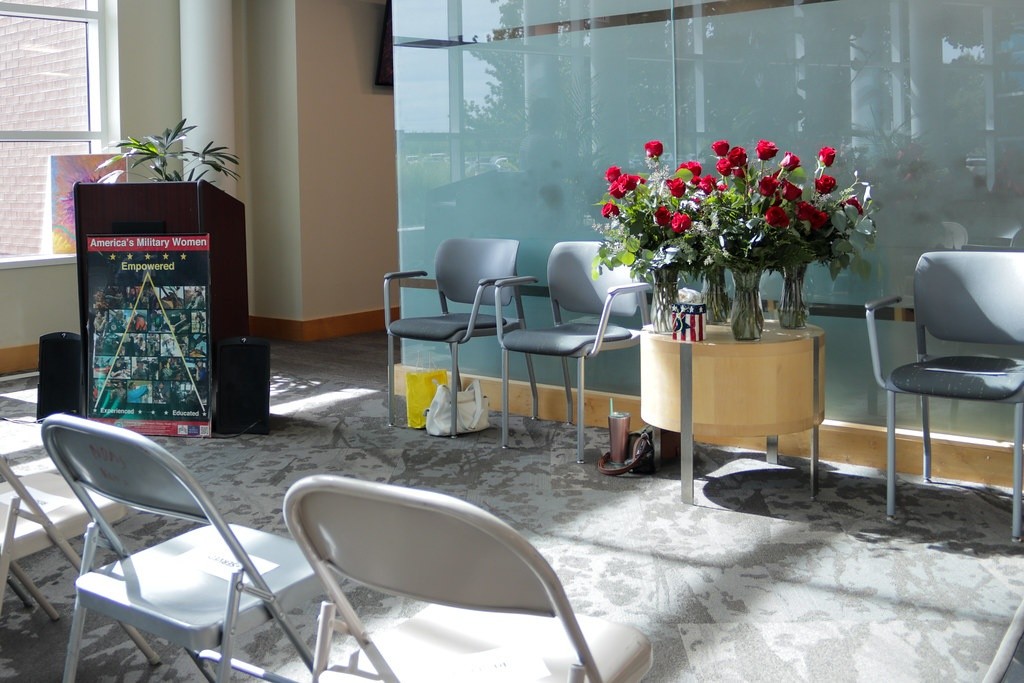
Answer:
[607,412,630,463]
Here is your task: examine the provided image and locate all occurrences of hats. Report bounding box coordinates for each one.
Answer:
[115,358,125,364]
[134,314,146,320]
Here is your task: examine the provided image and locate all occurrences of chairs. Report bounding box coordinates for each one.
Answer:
[0,412,655,683]
[864,250,1024,544]
[494,240,644,465]
[384,238,539,447]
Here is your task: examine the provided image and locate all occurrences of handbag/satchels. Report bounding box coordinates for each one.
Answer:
[425,380,491,436]
[405,349,448,428]
[598,424,680,476]
[671,303,707,342]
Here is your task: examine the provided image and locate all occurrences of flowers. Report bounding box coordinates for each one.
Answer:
[600,141,875,284]
[702,265,729,325]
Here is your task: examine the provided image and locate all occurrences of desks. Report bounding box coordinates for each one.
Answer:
[637,325,828,506]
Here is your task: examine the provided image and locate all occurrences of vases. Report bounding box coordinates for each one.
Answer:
[650,267,680,333]
[730,268,764,340]
[778,268,807,327]
